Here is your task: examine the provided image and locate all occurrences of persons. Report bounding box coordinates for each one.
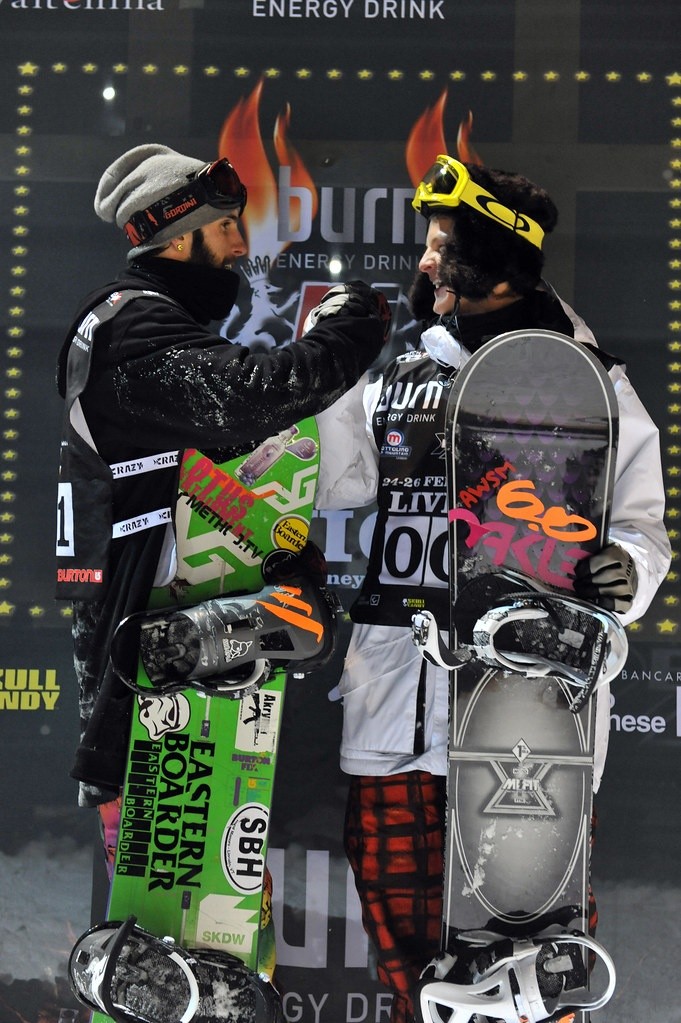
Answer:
[55,144,392,1023]
[311,152,674,1023]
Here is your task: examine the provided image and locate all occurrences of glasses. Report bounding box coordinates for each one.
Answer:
[194,157,248,219]
[411,154,471,214]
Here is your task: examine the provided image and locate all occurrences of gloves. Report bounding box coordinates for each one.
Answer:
[319,278,394,346]
[572,541,639,614]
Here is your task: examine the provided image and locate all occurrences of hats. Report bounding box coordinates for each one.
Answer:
[93,143,238,262]
[405,164,559,321]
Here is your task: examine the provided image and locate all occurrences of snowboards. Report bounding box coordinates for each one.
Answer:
[86,406,326,1023]
[431,326,620,1023]
